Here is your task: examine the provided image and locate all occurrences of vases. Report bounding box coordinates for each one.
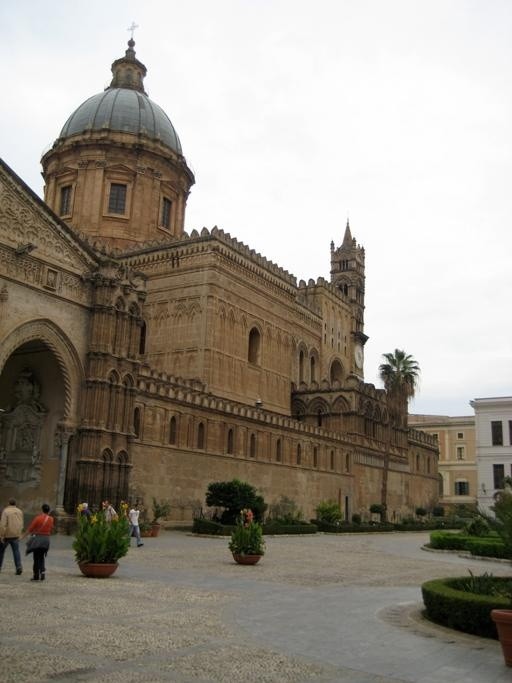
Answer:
[231,553,261,565]
[79,563,119,578]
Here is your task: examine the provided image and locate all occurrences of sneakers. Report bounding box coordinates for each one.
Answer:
[40,572,45,580]
[31,578,38,581]
[15,568,22,575]
[138,544,143,547]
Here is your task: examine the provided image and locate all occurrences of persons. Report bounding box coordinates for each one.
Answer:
[80,502,93,520]
[127,503,144,547]
[1,497,24,575]
[14,503,55,581]
[104,499,119,526]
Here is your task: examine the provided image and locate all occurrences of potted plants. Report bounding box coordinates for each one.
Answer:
[140,497,172,537]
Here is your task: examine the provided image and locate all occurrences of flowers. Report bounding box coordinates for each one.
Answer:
[72,504,131,566]
[228,507,267,556]
